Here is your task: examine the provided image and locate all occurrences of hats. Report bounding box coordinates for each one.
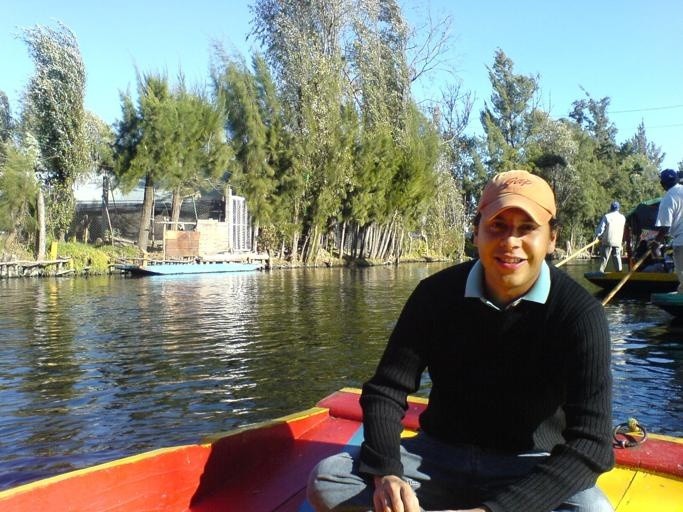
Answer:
[659,169,678,184]
[475,169,558,227]
[610,201,621,211]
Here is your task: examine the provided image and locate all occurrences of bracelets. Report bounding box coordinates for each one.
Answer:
[652,239,661,245]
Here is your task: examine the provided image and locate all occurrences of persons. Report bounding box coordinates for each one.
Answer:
[658,241,668,257]
[305,168,619,512]
[593,200,626,273]
[632,239,669,273]
[648,168,682,294]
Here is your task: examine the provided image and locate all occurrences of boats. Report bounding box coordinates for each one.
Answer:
[109,263,264,275]
[584,198,683,315]
